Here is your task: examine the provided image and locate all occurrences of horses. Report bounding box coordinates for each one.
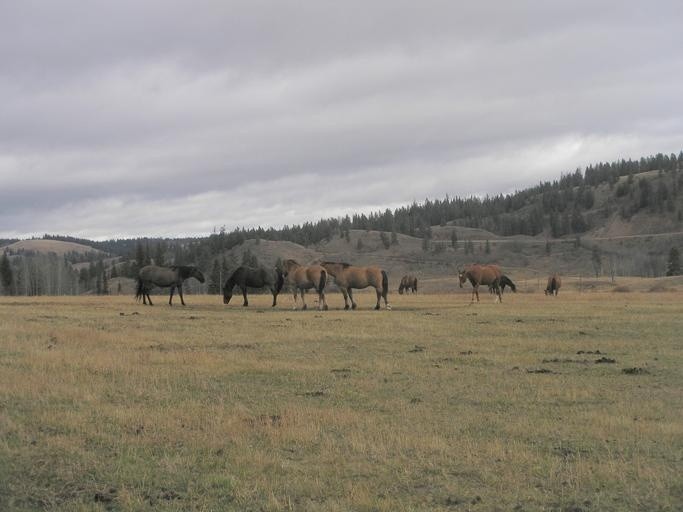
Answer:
[281,259,328,311]
[458,264,502,304]
[399,275,417,295]
[318,259,392,311]
[135,265,205,307]
[544,273,561,296]
[488,274,516,294]
[223,266,284,307]
